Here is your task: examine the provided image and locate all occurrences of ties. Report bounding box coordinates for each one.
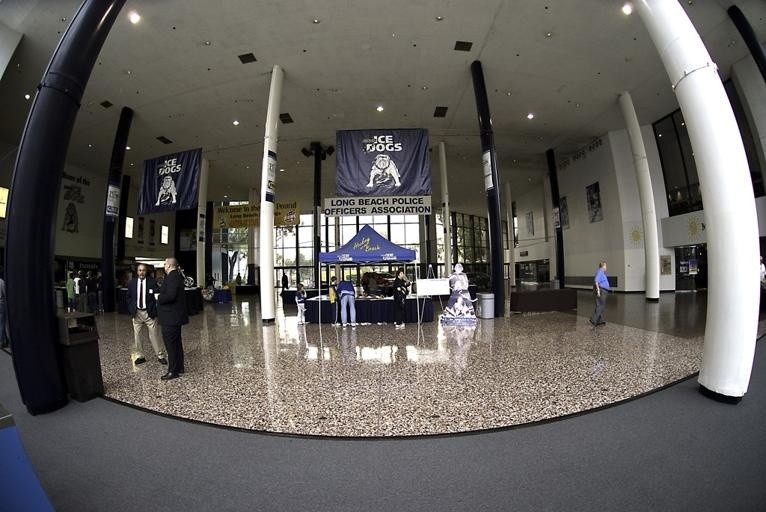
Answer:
[140,281,143,309]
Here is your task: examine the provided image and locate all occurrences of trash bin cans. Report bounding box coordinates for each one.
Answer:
[57,311,105,403]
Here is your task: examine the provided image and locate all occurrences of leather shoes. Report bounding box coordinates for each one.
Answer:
[161,368,184,379]
[158,359,167,364]
[135,358,145,364]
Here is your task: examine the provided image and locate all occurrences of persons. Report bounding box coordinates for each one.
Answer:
[126,264,168,365]
[0,278,8,348]
[369,274,377,291]
[443,264,475,318]
[329,276,341,326]
[589,262,610,327]
[67,273,104,313]
[154,257,189,380]
[360,273,369,291]
[295,284,307,325]
[392,270,410,328]
[337,281,357,327]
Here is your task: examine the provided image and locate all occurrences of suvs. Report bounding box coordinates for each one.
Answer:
[360,271,408,292]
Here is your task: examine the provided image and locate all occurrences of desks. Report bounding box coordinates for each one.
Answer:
[280,287,329,304]
[115,286,204,317]
[212,289,232,303]
[510,287,577,312]
[235,285,257,295]
[304,294,434,323]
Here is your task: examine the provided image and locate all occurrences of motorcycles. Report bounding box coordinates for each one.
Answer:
[154,264,196,289]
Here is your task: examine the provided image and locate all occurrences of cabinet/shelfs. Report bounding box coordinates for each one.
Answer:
[67,255,102,279]
